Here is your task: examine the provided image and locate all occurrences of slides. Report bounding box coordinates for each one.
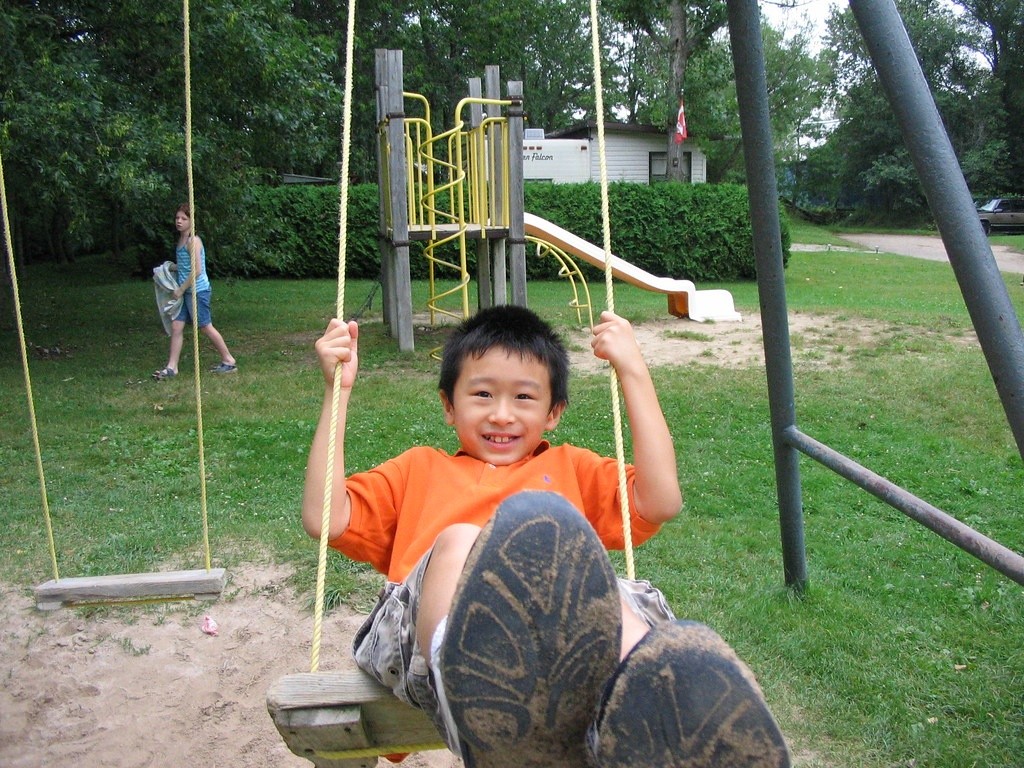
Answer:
[523,211,696,294]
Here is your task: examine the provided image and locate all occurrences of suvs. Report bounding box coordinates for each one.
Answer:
[977,198,1024,236]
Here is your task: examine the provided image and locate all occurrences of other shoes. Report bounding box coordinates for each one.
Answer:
[599,619,792,768]
[440,490,624,768]
[211,362,237,373]
[152,367,174,378]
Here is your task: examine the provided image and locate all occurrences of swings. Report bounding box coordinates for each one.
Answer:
[0,1,230,609]
[262,0,639,761]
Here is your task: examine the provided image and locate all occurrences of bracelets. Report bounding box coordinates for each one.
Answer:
[181,285,186,291]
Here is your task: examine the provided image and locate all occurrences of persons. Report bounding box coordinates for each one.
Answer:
[299,319,792,768]
[151,203,240,379]
